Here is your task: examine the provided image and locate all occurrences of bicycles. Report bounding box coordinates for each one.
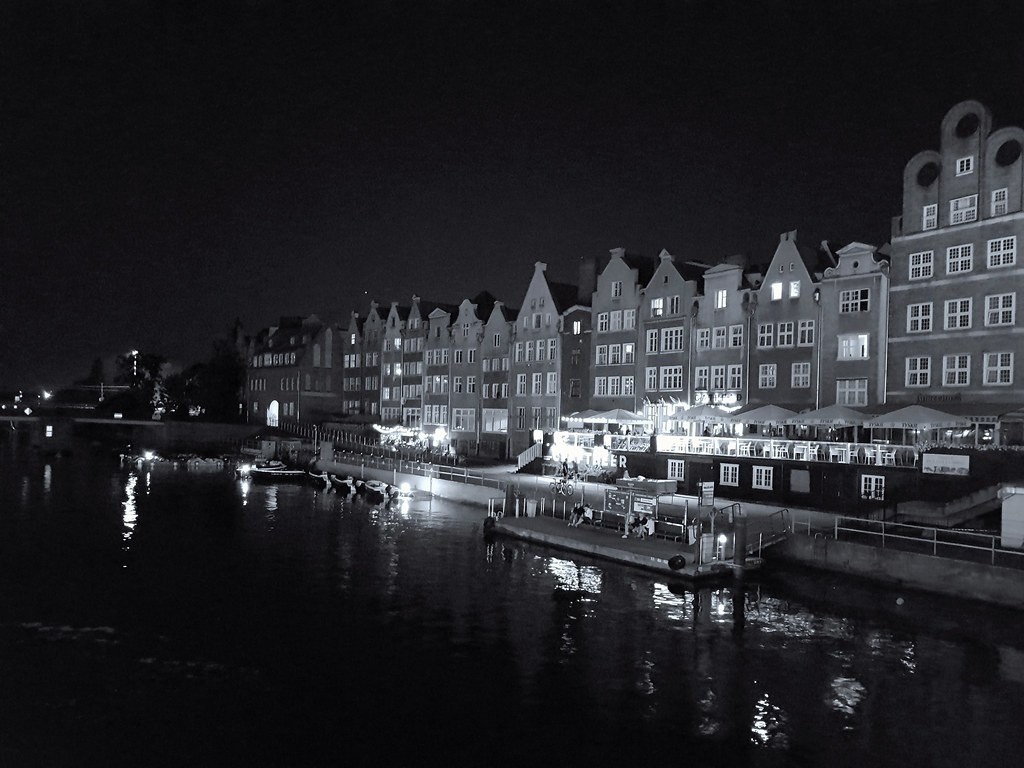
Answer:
[549,478,574,496]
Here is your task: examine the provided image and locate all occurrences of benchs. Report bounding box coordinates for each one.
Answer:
[569,507,683,543]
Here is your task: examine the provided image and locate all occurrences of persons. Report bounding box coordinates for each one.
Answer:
[567,502,655,540]
[569,461,578,478]
[563,457,568,479]
[617,425,623,435]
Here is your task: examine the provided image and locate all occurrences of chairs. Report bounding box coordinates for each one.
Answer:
[863,446,899,466]
[763,442,793,459]
[794,444,827,461]
[727,442,759,457]
[828,443,862,464]
[679,439,723,455]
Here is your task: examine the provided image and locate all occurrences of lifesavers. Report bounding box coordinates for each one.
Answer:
[668,554,685,570]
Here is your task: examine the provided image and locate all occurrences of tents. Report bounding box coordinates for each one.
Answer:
[569,404,978,444]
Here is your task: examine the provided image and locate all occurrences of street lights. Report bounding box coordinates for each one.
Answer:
[313,425,317,454]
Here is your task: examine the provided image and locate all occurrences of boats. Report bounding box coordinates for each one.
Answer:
[254,459,399,500]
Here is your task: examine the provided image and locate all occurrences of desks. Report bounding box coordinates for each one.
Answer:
[736,443,747,456]
[871,450,888,466]
[767,445,785,458]
[835,448,850,463]
[681,441,691,453]
[797,446,814,460]
[698,442,711,454]
